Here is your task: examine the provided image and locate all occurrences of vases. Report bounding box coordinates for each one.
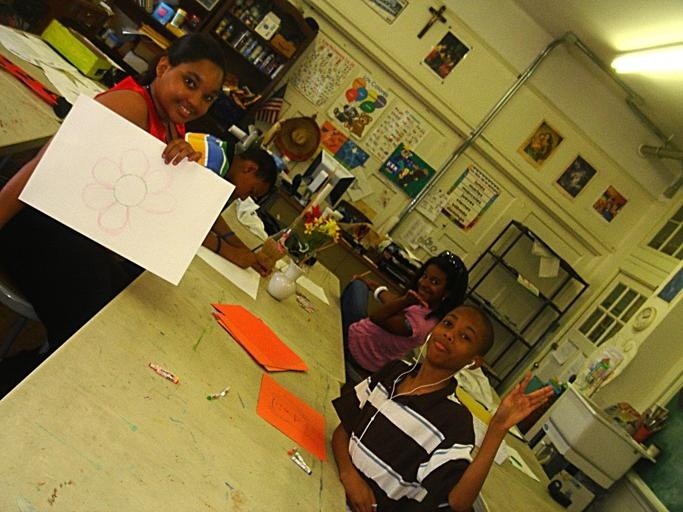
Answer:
[266,263,306,303]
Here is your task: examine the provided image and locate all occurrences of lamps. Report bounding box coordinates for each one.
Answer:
[609,39,683,78]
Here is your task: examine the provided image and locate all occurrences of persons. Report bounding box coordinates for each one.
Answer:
[340,249,468,375]
[183,128,277,277]
[330,304,554,512]
[529,132,551,161]
[1,30,227,353]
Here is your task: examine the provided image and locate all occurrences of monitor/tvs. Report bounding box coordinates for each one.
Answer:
[294,149,356,210]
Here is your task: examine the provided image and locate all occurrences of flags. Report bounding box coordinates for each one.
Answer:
[253,83,287,125]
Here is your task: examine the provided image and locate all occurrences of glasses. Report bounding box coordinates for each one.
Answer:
[442,249,464,274]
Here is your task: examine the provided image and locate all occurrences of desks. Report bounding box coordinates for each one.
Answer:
[2,210,356,512]
[1,18,112,168]
[411,328,568,511]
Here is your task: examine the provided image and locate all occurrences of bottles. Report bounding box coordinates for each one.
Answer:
[215,18,285,78]
[228,0,264,28]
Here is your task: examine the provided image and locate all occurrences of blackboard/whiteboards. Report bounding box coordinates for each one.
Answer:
[627,372,682,512]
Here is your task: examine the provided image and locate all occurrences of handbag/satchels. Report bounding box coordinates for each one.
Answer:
[260,210,306,255]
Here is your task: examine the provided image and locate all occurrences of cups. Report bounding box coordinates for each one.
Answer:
[255,237,286,271]
[647,443,660,458]
[634,424,649,443]
[170,8,187,29]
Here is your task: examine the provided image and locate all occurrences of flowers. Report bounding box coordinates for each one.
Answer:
[294,202,341,270]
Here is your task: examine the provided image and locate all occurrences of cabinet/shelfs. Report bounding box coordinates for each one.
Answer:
[28,0,230,100]
[451,218,590,392]
[196,0,320,147]
[265,187,405,321]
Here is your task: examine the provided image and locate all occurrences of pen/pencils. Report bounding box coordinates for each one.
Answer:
[643,406,668,432]
[206,386,231,401]
[510,456,523,468]
[250,242,264,252]
[148,363,182,385]
[459,386,488,412]
[287,447,313,475]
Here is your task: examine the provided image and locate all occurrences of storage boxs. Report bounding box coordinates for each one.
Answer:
[547,381,658,482]
[38,19,112,82]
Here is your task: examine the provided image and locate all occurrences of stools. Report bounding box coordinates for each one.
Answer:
[0,282,42,357]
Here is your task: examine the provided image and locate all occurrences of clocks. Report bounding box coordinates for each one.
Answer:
[632,305,658,334]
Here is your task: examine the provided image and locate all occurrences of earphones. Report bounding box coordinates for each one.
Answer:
[463,360,475,369]
[425,331,432,342]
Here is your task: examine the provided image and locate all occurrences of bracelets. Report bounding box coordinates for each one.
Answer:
[221,231,235,240]
[214,234,222,253]
[374,286,388,302]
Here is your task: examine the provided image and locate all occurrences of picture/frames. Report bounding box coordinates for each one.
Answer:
[514,114,566,174]
[419,29,473,82]
[550,150,600,205]
[587,182,629,226]
